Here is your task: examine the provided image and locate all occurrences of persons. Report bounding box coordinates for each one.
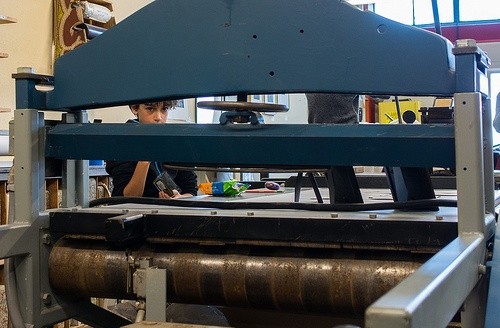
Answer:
[493,92,500,134]
[104,98,199,201]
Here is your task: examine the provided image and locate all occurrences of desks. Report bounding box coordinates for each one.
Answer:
[195,100,289,179]
